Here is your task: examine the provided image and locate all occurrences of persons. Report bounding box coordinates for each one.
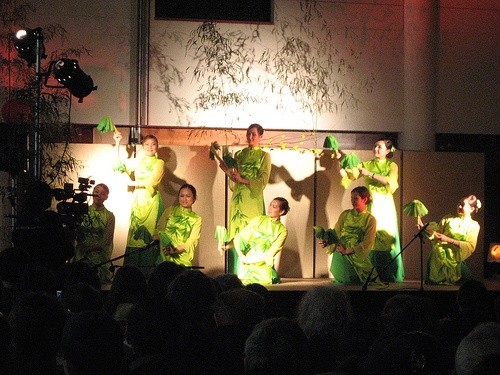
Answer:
[220,196,290,284]
[319,186,381,284]
[332,138,406,281]
[113,131,165,265]
[415,195,482,286]
[73,184,115,286]
[10,181,77,265]
[0,247,500,375]
[219,124,272,274]
[152,184,201,270]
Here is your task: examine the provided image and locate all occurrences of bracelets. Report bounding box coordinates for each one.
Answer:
[372,173,375,179]
[451,239,455,246]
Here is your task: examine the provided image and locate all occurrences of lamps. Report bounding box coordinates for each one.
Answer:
[14,28,48,68]
[55,58,98,103]
